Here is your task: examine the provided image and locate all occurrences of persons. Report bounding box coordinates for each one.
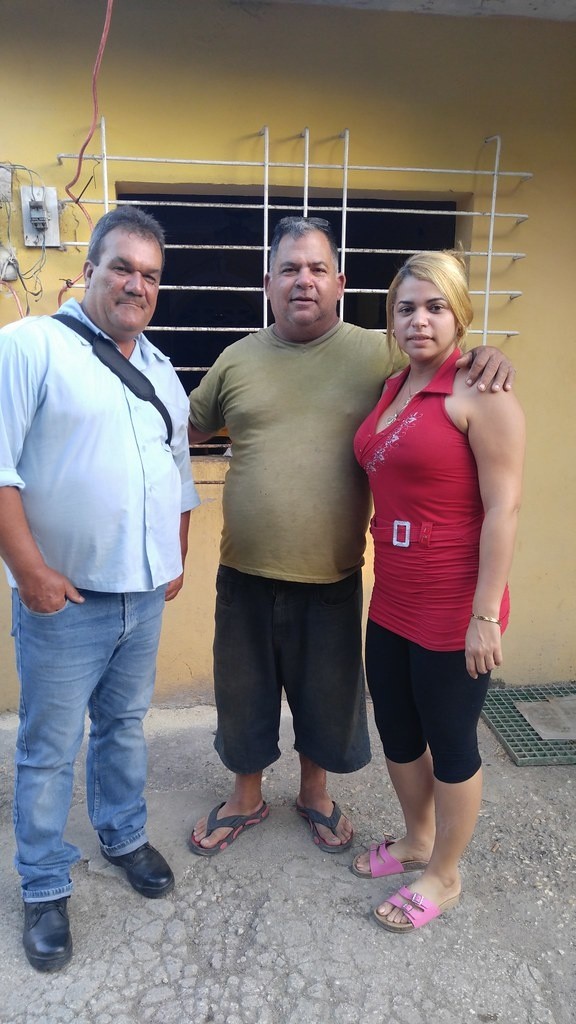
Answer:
[185,215,513,857]
[352,249,524,934]
[0,204,201,972]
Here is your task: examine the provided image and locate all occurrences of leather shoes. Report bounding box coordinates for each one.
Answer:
[21,897,73,969]
[101,839,174,896]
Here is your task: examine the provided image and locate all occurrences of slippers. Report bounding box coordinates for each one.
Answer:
[352,840,429,878]
[295,795,355,853]
[189,800,270,855]
[373,887,458,933]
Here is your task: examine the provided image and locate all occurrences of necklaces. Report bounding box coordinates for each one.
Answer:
[386,371,420,427]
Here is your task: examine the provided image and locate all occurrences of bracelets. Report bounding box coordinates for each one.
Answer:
[470,612,500,625]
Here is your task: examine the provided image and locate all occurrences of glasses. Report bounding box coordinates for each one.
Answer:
[281,215,331,227]
